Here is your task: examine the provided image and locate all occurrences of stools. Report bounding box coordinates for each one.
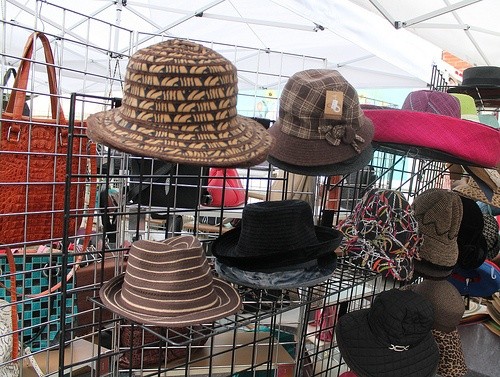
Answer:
[131,328,297,377]
[16,335,111,377]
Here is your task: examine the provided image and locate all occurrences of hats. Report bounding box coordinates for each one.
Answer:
[476,211,499,258]
[209,197,343,271]
[97,234,240,326]
[401,90,462,121]
[335,286,439,376]
[336,186,415,281]
[441,93,479,122]
[212,260,338,291]
[271,66,375,175]
[443,66,499,106]
[454,166,500,210]
[457,198,486,272]
[113,317,208,367]
[412,188,457,280]
[403,278,467,377]
[88,39,276,168]
[484,292,500,337]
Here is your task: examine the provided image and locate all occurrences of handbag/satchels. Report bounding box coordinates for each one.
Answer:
[345,165,379,210]
[1,68,30,116]
[267,172,314,215]
[206,168,251,207]
[0,298,18,377]
[1,28,92,238]
[127,149,207,206]
[0,247,75,346]
